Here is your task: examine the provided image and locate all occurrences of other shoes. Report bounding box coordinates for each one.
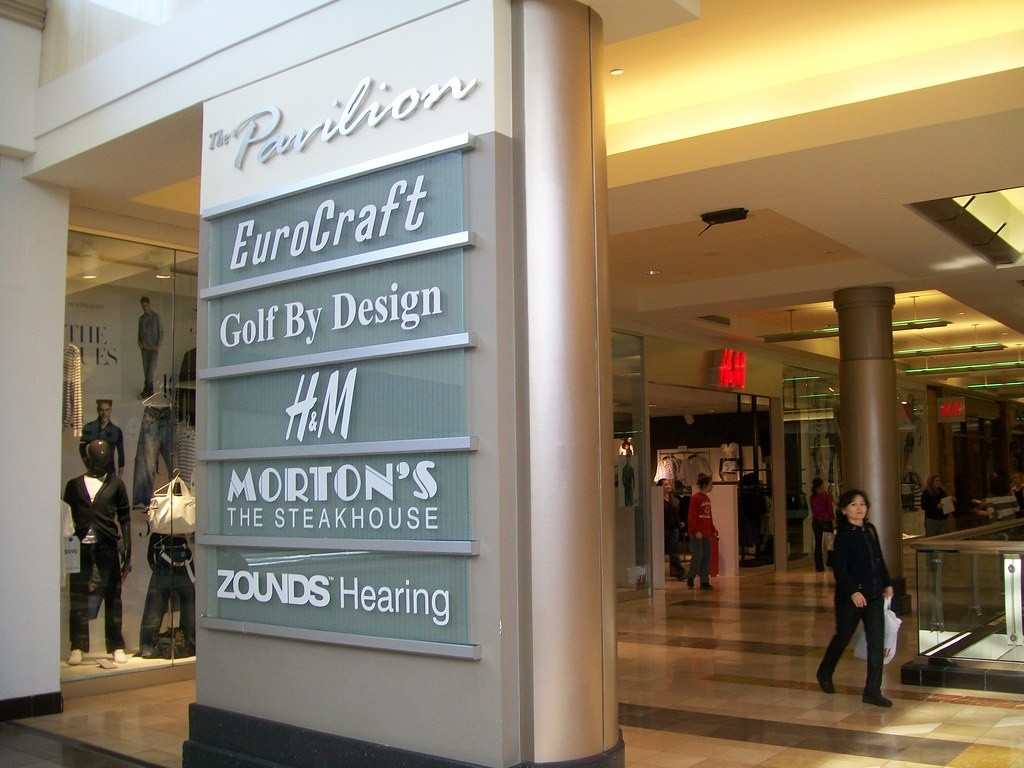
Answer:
[686,578,694,588]
[700,582,714,589]
[677,571,689,581]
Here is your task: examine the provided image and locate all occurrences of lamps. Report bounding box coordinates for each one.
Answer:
[756,296,1024,401]
[154,252,173,280]
[75,239,103,280]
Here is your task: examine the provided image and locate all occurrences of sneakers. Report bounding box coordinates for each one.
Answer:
[817,666,837,693]
[862,690,893,707]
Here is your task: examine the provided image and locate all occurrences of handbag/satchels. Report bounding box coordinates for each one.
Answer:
[822,532,836,552]
[157,545,194,577]
[853,595,902,665]
[148,476,196,534]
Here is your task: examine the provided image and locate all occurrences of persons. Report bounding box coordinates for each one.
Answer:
[976,477,1020,523]
[809,477,835,572]
[622,455,636,506]
[687,476,719,589]
[146,532,180,612]
[64,440,131,664]
[79,399,125,477]
[816,489,893,707]
[137,297,163,400]
[920,473,957,537]
[657,478,688,580]
[1011,471,1024,519]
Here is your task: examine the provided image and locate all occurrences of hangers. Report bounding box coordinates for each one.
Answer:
[141,378,177,407]
[661,451,701,459]
[153,468,193,498]
